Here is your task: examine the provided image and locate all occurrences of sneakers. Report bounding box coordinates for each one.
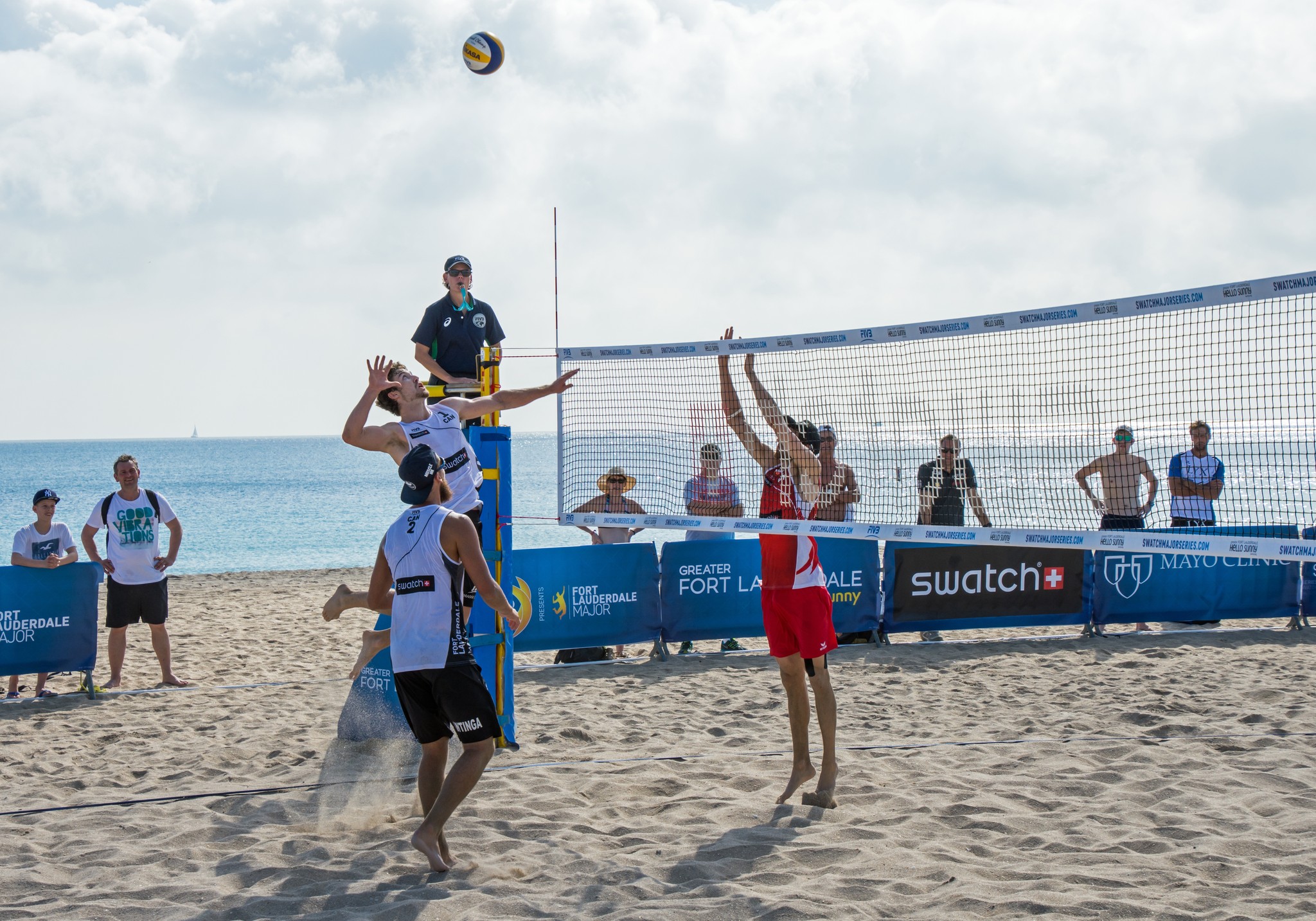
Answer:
[719,638,748,651]
[920,631,943,641]
[678,641,693,654]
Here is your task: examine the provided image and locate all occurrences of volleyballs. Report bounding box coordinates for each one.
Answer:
[462,31,505,76]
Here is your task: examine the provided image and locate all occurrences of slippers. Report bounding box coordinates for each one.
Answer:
[46,670,94,680]
[5,692,20,699]
[17,684,36,692]
[34,690,58,697]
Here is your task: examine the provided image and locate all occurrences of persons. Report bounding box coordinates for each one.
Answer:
[81,455,189,690]
[914,435,993,642]
[5,490,79,700]
[322,354,580,680]
[718,327,838,805]
[571,467,648,657]
[1167,420,1225,527]
[411,256,505,428]
[811,424,860,522]
[678,443,748,654]
[367,443,521,871]
[1075,425,1158,631]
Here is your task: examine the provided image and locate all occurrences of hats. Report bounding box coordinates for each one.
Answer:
[398,443,437,505]
[817,425,836,438]
[597,467,636,494]
[1114,425,1134,436]
[700,444,721,456]
[782,414,820,455]
[32,488,60,505]
[444,255,472,271]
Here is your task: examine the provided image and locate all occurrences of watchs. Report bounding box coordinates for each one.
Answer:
[1146,501,1154,506]
[827,489,833,497]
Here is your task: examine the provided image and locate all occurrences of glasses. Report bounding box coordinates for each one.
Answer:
[820,436,836,443]
[445,269,472,277]
[607,478,625,484]
[433,456,446,475]
[701,453,721,460]
[1114,435,1133,442]
[939,447,958,454]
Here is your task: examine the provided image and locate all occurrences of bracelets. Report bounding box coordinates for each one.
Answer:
[591,530,596,535]
[629,529,635,535]
[983,523,992,527]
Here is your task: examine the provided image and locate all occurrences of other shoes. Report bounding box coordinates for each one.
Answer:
[0,687,5,694]
